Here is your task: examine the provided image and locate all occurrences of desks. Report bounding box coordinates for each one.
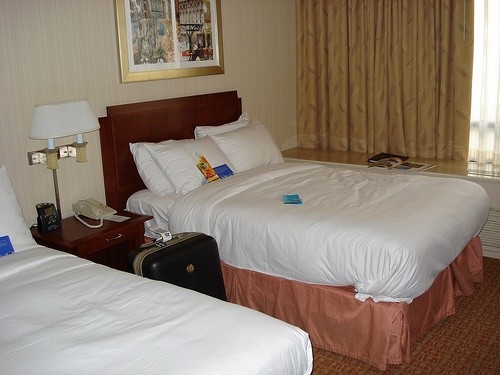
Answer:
[280,147,500,261]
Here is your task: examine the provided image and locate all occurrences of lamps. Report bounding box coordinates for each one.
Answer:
[26,97,102,220]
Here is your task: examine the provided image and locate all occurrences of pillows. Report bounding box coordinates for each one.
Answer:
[129,139,193,198]
[194,111,250,139]
[143,135,237,196]
[210,118,285,172]
[0,164,41,257]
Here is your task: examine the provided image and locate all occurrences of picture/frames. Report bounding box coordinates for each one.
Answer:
[114,0,225,84]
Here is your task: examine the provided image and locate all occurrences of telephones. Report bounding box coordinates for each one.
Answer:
[72,197,117,220]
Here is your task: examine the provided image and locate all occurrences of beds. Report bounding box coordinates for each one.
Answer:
[0,164,314,375]
[97,90,491,372]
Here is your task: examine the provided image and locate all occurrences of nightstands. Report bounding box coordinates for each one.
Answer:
[29,207,154,276]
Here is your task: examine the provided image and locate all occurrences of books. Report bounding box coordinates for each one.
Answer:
[196,153,220,183]
[368,158,436,172]
[367,153,411,163]
